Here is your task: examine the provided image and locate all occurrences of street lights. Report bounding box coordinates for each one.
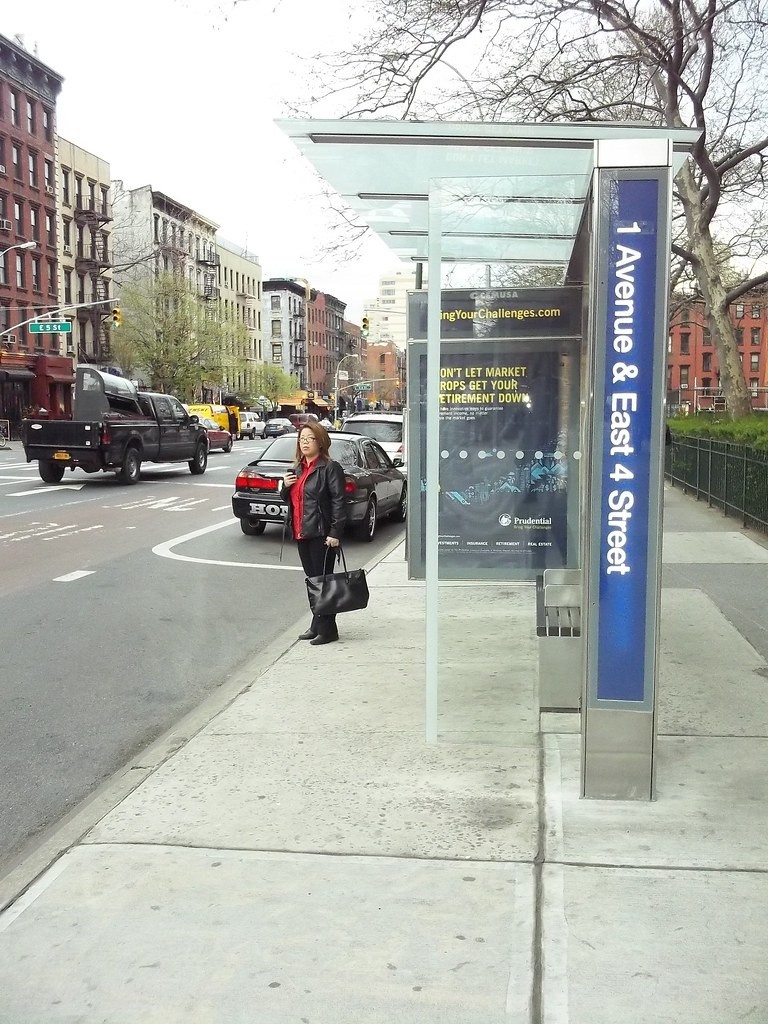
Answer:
[362,317,369,331]
[332,354,359,420]
[373,352,392,392]
[0,241,37,257]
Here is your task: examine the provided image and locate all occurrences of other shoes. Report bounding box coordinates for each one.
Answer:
[298,628,319,640]
[310,633,339,645]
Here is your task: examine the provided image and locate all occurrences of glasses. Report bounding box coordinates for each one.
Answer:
[298,436,316,443]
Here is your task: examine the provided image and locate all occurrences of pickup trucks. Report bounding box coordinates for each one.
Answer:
[21,393,208,485]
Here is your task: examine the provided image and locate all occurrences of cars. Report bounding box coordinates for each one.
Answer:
[232,430,407,542]
[240,412,319,440]
[199,418,232,454]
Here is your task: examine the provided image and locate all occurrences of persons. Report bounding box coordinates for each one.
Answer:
[229,410,238,441]
[280,420,347,645]
[319,412,334,422]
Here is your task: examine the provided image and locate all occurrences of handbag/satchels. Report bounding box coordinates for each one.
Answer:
[304,544,370,616]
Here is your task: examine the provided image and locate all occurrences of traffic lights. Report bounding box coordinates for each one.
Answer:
[113,308,121,324]
[396,381,399,389]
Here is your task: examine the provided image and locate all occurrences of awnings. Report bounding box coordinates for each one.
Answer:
[279,396,352,410]
[0,369,78,382]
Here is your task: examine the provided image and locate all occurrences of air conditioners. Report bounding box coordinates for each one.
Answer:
[0,219,12,231]
[0,165,6,173]
[46,185,54,194]
[65,245,71,252]
[68,345,76,352]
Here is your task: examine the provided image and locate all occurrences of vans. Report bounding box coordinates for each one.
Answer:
[339,414,406,479]
